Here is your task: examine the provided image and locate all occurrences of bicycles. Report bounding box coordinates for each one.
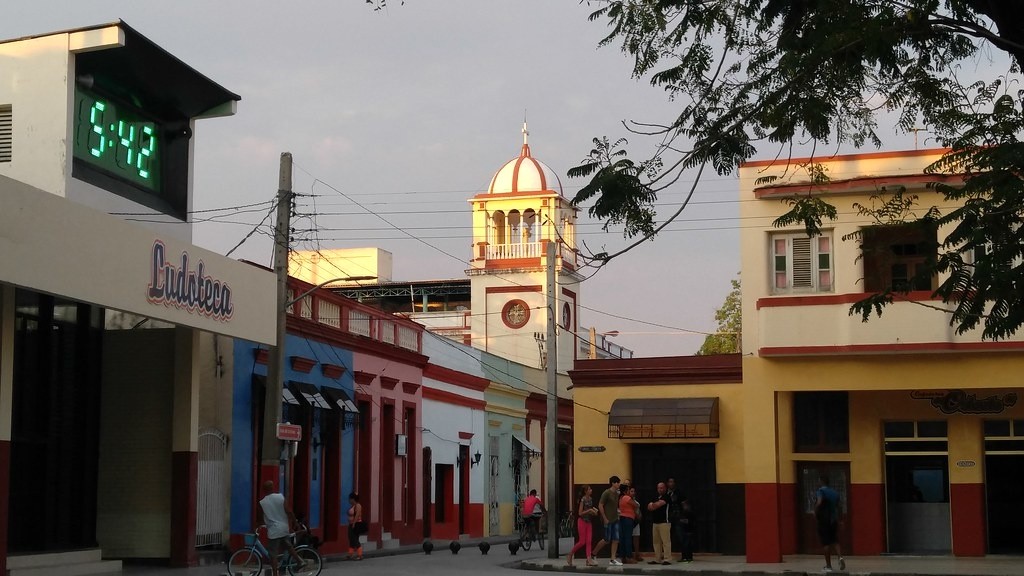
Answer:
[520,513,545,551]
[227,521,323,576]
[560,509,576,538]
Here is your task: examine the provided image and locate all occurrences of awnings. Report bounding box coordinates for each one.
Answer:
[321,386,359,413]
[252,373,299,405]
[512,434,543,458]
[607,397,719,438]
[292,380,332,409]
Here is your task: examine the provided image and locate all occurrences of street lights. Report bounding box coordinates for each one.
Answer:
[257,274,379,564]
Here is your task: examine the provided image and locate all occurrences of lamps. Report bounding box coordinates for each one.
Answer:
[602,330,619,337]
[457,449,467,468]
[471,450,481,469]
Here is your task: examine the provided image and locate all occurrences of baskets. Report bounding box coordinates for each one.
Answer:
[244,533,256,546]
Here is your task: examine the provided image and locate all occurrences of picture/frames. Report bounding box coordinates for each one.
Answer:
[397,434,408,455]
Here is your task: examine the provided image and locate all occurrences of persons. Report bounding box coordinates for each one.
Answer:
[616,479,645,564]
[566,485,599,566]
[647,478,694,565]
[342,493,363,561]
[813,475,845,571]
[591,476,623,566]
[522,489,546,547]
[258,481,306,576]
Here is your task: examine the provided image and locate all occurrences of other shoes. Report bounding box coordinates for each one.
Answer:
[687,559,693,562]
[677,558,689,563]
[838,556,846,570]
[355,556,364,561]
[647,561,661,564]
[586,559,598,566]
[635,556,643,561]
[622,556,637,564]
[591,552,596,561]
[344,555,355,560]
[821,566,832,572]
[609,559,623,566]
[566,554,572,565]
[662,562,671,565]
[294,561,307,571]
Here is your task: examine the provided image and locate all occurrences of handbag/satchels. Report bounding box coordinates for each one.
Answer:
[355,520,368,536]
